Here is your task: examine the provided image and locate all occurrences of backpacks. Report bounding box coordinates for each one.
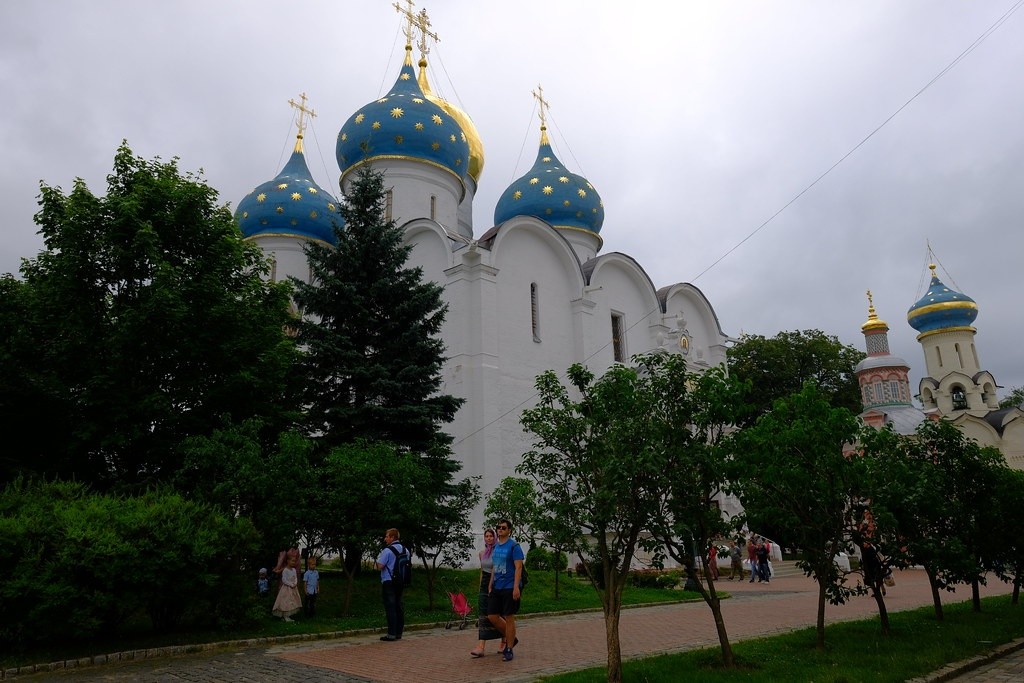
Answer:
[511,543,528,590]
[383,545,412,588]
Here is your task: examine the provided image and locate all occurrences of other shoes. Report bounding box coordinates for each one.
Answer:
[513,637,519,648]
[498,642,507,653]
[380,636,394,641]
[471,646,485,657]
[750,578,770,584]
[503,648,514,662]
[283,617,295,622]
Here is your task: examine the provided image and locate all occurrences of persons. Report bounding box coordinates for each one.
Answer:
[707,532,775,584]
[486,517,524,662]
[253,567,271,598]
[470,528,507,657]
[272,548,302,623]
[376,528,410,641]
[302,556,319,618]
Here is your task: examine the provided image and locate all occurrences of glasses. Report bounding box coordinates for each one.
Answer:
[496,524,509,530]
